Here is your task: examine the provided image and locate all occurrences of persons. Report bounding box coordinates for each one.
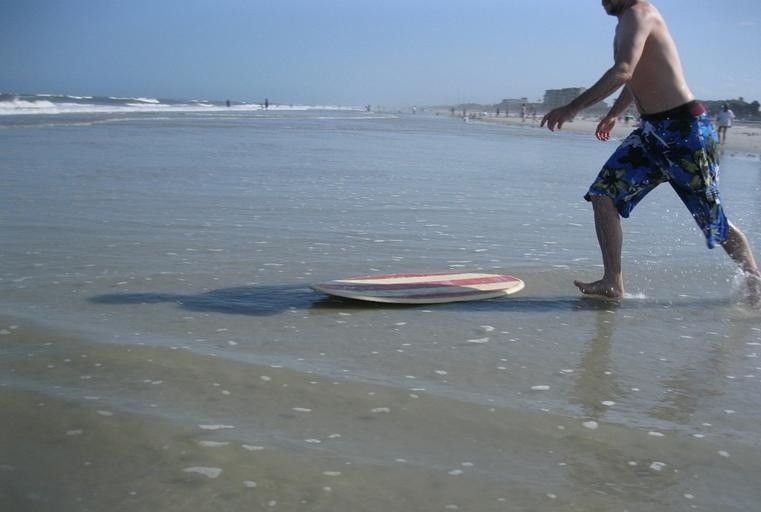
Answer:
[521,104,526,122]
[716,103,736,144]
[539,1,760,307]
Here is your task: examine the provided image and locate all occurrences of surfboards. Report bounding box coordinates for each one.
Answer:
[309,272,526,305]
